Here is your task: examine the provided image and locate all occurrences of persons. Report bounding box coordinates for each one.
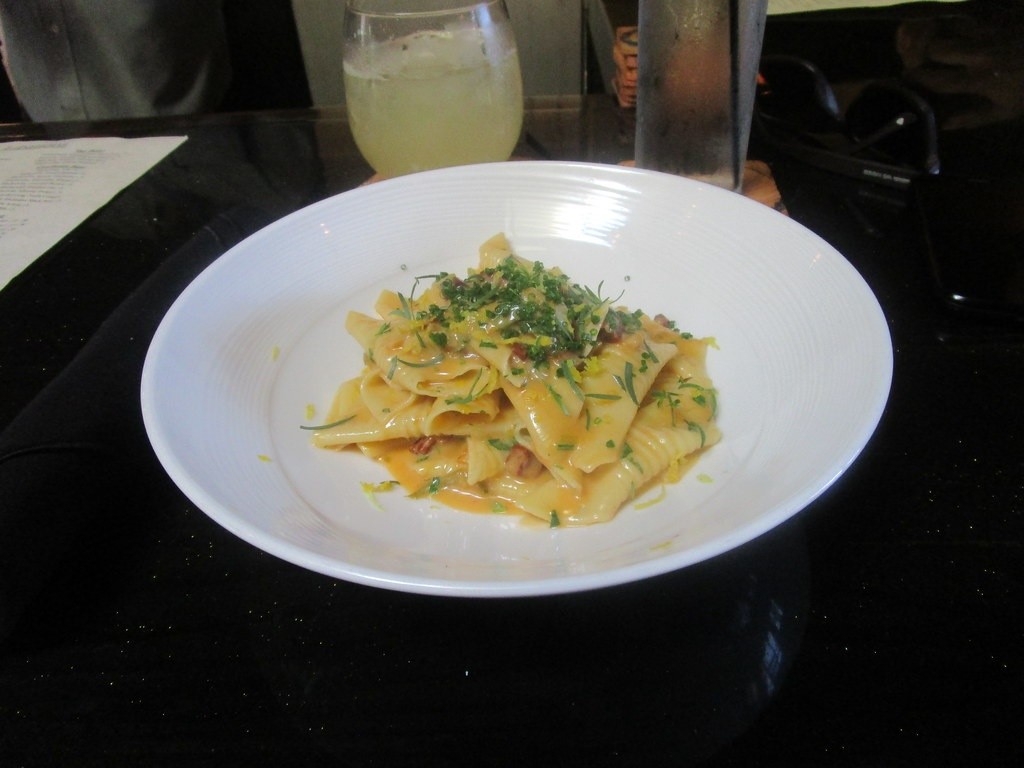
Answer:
[777,61,930,163]
[0,0,225,125]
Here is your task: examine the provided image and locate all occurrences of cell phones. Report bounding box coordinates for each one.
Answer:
[913,169,1024,320]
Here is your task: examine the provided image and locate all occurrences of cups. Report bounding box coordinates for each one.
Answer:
[341,0,524,180]
[634,0,770,197]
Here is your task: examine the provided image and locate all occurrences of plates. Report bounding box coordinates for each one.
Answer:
[140,160,894,599]
